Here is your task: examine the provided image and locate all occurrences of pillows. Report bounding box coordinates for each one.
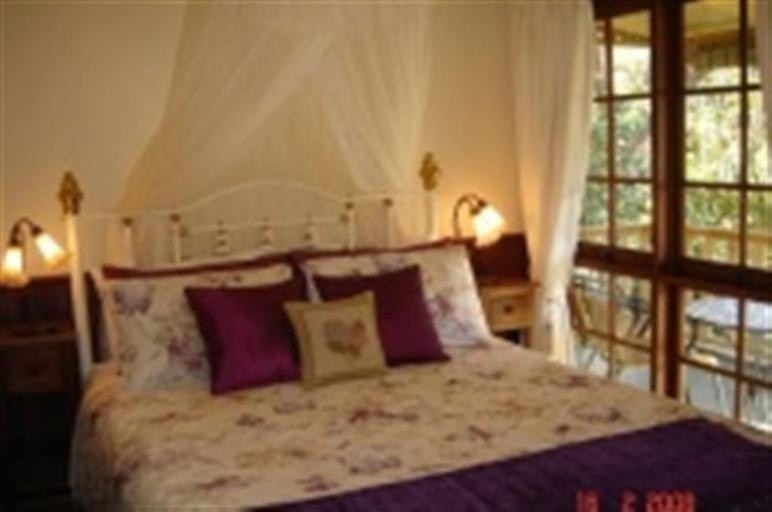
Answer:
[290,238,496,349]
[182,277,313,396]
[93,243,299,390]
[283,289,392,390]
[311,262,452,364]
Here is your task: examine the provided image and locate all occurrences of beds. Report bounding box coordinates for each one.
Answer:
[44,152,772,512]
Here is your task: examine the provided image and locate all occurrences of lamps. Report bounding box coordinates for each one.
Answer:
[452,191,503,241]
[0,217,64,289]
